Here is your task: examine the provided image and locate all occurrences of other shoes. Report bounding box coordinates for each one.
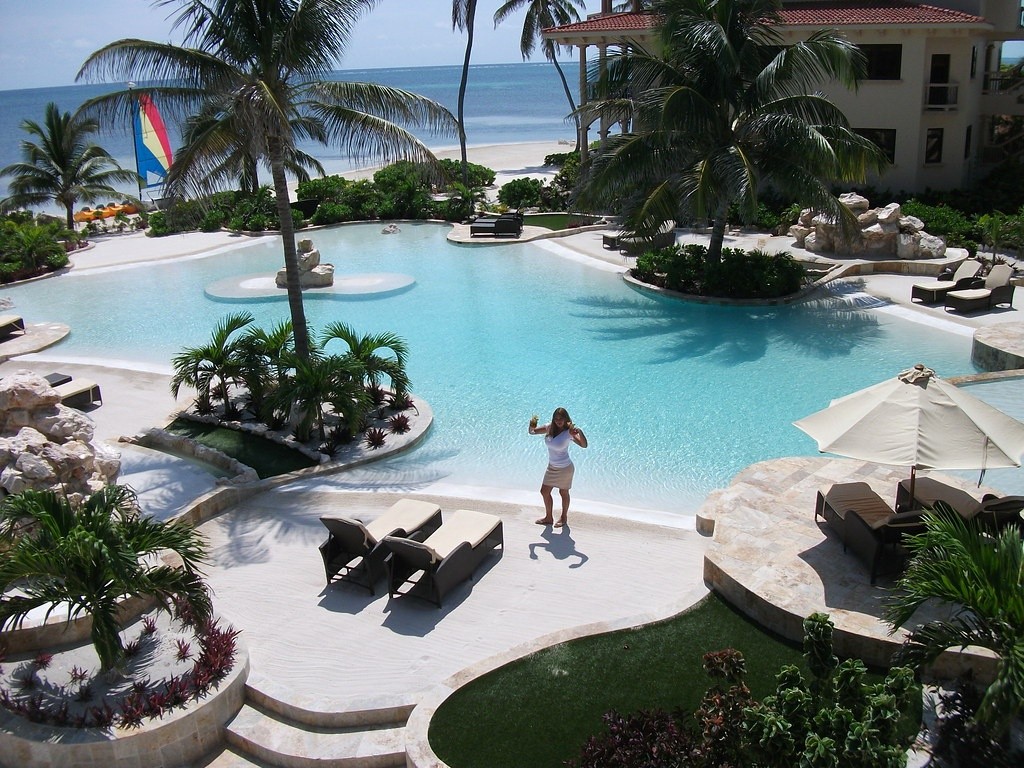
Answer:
[554,519,567,527]
[535,517,553,524]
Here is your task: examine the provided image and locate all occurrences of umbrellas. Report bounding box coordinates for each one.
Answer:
[792,364,1024,510]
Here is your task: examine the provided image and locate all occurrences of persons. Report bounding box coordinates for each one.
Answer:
[529,407,587,527]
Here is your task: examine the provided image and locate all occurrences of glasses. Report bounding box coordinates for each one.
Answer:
[553,417,564,422]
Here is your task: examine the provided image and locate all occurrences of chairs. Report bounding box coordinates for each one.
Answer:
[470,207,524,239]
[603,214,677,257]
[0,316,26,344]
[910,259,1016,313]
[815,477,1024,583]
[42,372,103,412]
[317,498,503,608]
